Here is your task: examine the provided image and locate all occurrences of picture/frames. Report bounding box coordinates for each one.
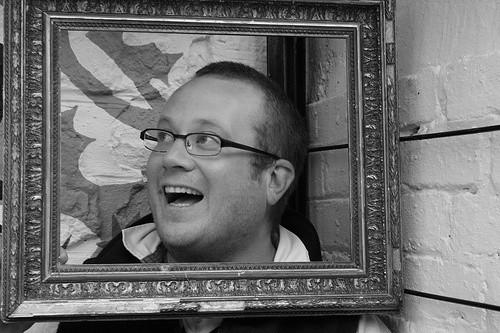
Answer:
[4,0,402,320]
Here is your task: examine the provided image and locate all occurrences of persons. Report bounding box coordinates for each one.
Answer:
[0,61,392,333]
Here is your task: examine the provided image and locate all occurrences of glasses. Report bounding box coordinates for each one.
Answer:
[139,128,280,159]
